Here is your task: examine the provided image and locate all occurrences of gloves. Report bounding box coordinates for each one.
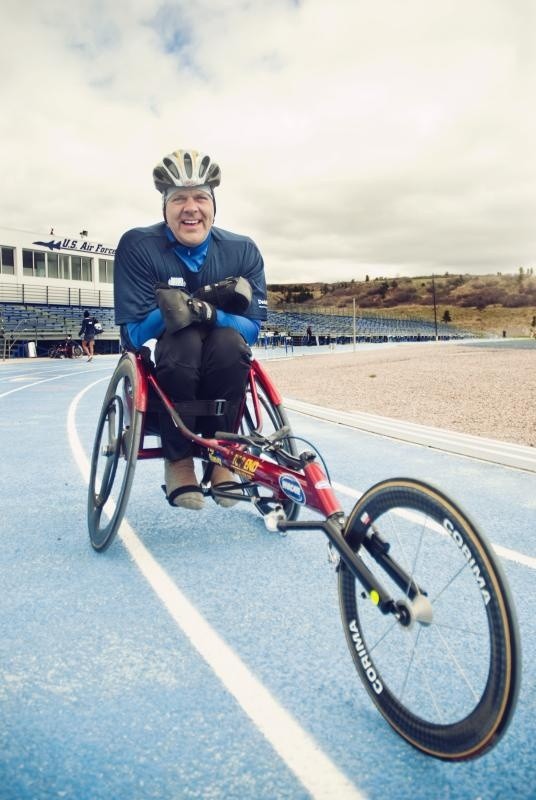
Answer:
[152,282,217,334]
[79,332,81,337]
[200,276,252,315]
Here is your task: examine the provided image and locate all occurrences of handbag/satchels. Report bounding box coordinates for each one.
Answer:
[93,316,105,334]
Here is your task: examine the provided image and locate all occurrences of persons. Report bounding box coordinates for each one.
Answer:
[114,149,268,509]
[79,311,95,361]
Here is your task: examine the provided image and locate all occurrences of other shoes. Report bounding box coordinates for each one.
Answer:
[164,457,205,510]
[87,356,92,362]
[209,463,244,507]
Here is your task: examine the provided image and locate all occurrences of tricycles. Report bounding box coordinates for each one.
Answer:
[83,328,521,762]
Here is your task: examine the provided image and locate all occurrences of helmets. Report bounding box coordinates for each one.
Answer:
[153,148,221,193]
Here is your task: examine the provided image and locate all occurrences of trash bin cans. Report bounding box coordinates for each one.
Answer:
[24,342,37,358]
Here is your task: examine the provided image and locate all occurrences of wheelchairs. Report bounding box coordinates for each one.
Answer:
[51,343,83,358]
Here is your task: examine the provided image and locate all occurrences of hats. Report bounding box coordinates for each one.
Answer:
[84,310,90,318]
